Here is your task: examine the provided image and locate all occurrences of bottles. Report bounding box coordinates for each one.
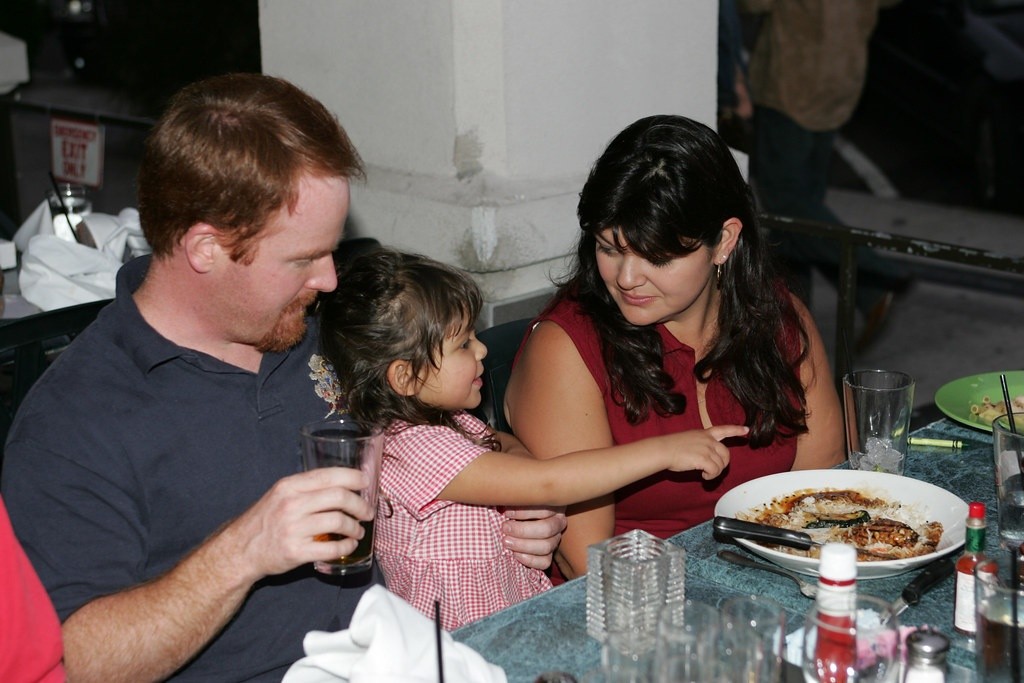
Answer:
[951,501,996,637]
[812,542,856,683]
[903,628,950,683]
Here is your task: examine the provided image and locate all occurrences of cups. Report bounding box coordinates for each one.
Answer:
[605,597,785,683]
[843,368,916,476]
[975,556,1024,683]
[298,419,383,575]
[48,182,86,242]
[991,413,1024,543]
[802,595,903,683]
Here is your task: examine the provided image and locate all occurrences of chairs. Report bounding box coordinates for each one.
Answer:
[0,296,117,449]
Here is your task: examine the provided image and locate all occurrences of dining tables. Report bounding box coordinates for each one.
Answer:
[449,412,999,683]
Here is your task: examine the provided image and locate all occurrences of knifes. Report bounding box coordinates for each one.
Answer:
[893,559,952,611]
[713,514,899,560]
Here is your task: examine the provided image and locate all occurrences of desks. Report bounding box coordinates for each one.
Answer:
[0,248,43,321]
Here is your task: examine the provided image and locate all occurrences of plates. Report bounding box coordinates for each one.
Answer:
[714,468,971,577]
[935,371,1024,432]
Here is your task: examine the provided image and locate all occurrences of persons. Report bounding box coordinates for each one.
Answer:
[504,115,845,580]
[0,496,69,683]
[719,1,917,363]
[1,72,507,683]
[318,250,750,633]
